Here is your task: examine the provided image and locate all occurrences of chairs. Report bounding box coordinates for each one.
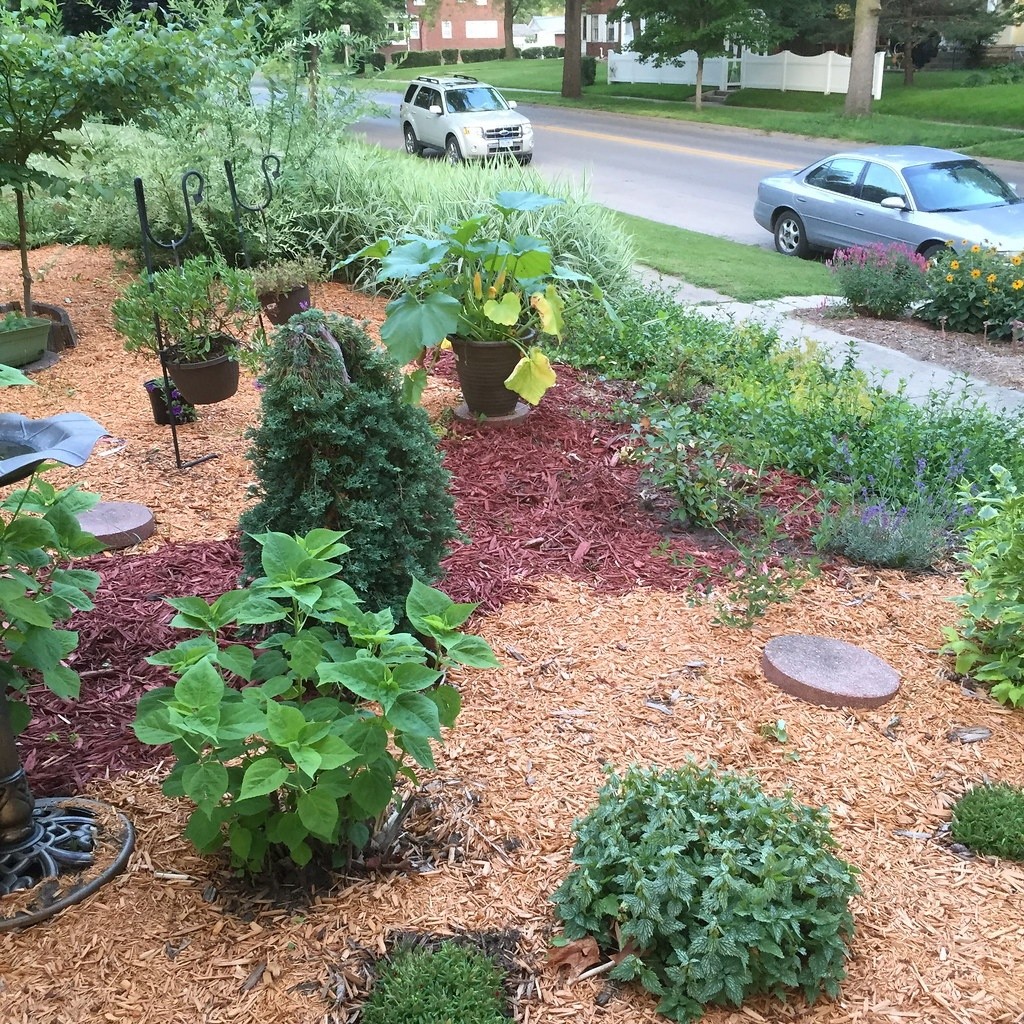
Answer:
[435,97,455,113]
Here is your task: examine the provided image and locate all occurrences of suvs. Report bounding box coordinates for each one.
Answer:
[399,73,535,167]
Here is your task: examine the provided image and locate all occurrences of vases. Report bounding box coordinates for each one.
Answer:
[143,377,196,424]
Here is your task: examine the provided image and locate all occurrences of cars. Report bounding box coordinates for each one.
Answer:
[752,143,1024,271]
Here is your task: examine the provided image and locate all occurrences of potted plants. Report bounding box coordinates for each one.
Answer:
[110,253,261,406]
[318,189,623,417]
[240,253,332,326]
[0,310,52,368]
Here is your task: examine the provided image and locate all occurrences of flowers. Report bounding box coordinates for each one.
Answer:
[147,377,200,422]
